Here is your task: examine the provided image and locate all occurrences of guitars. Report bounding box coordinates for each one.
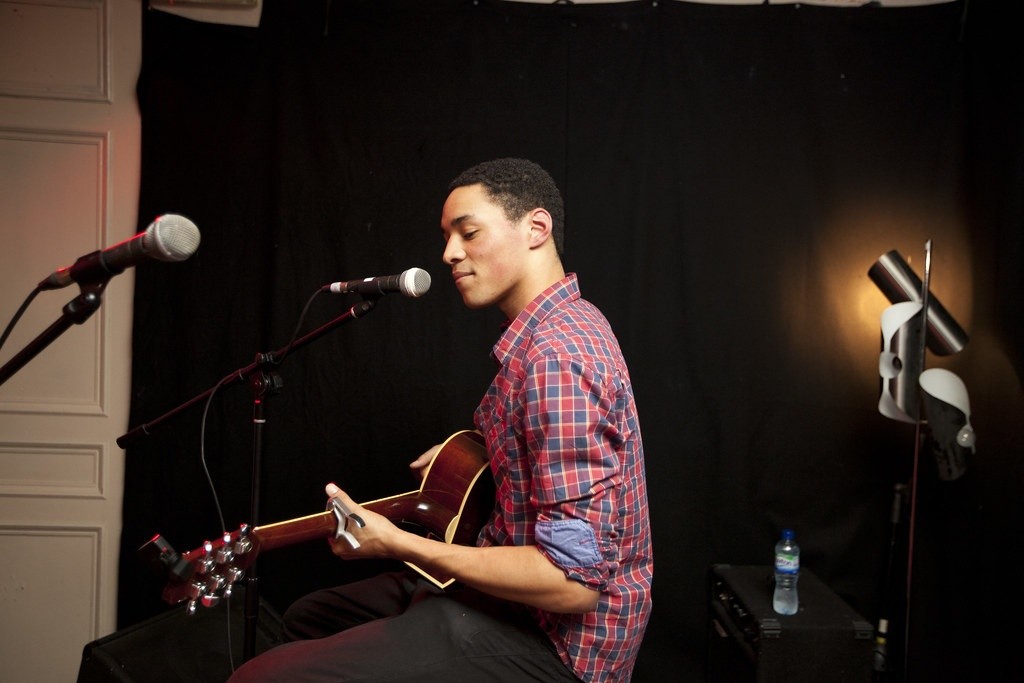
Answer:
[139,429,496,617]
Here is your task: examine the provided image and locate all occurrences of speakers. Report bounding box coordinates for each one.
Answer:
[77,585,281,683]
[707,562,875,683]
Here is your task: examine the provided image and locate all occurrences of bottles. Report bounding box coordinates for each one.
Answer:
[772,531,800,614]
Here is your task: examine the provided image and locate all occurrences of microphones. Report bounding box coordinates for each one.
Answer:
[37,213,200,290]
[320,267,433,298]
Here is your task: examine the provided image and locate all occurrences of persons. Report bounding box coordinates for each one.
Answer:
[223,159,652,683]
